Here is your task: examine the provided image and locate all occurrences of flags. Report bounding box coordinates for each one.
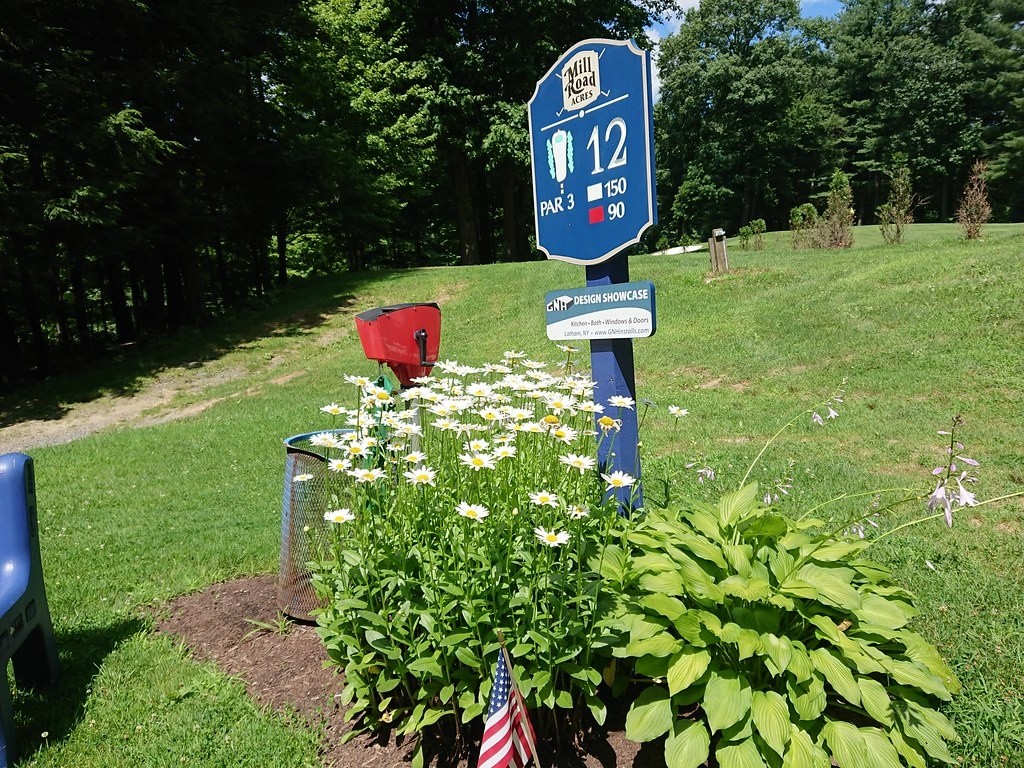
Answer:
[476,646,539,768]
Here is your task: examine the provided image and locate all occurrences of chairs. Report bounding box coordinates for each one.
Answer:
[0,452,63,768]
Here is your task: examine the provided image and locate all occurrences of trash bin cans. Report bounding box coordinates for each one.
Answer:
[273,427,373,624]
